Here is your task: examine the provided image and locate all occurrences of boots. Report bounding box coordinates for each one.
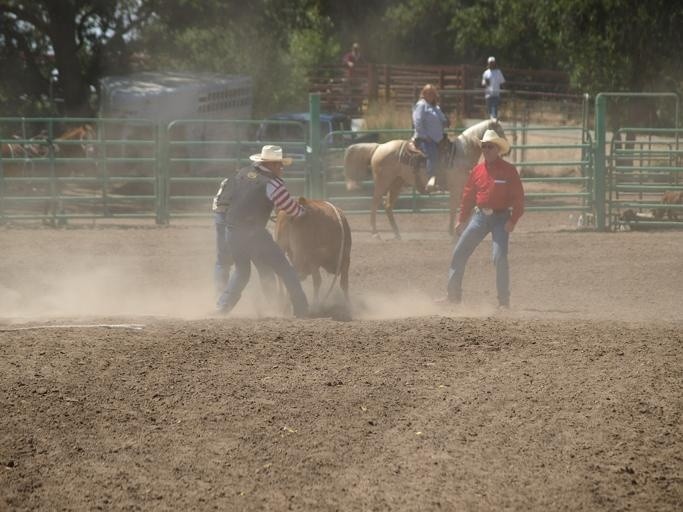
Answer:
[496,290,513,312]
[433,287,464,308]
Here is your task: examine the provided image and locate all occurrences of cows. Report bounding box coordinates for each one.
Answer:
[276,196,352,310]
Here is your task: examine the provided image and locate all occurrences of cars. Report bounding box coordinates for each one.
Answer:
[232,109,355,179]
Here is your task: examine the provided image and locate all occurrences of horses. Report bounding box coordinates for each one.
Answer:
[343,114,511,242]
[0,121,97,232]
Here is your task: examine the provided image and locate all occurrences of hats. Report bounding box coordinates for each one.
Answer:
[487,56,497,64]
[469,129,513,156]
[247,143,294,167]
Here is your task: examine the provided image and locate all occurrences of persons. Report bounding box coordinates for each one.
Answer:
[481,55,506,119]
[432,128,526,311]
[342,42,368,109]
[212,143,311,319]
[212,175,278,304]
[411,83,450,187]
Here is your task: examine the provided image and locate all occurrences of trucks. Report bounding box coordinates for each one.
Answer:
[84,70,253,189]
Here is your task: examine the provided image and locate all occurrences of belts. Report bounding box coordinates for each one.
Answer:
[474,206,513,218]
